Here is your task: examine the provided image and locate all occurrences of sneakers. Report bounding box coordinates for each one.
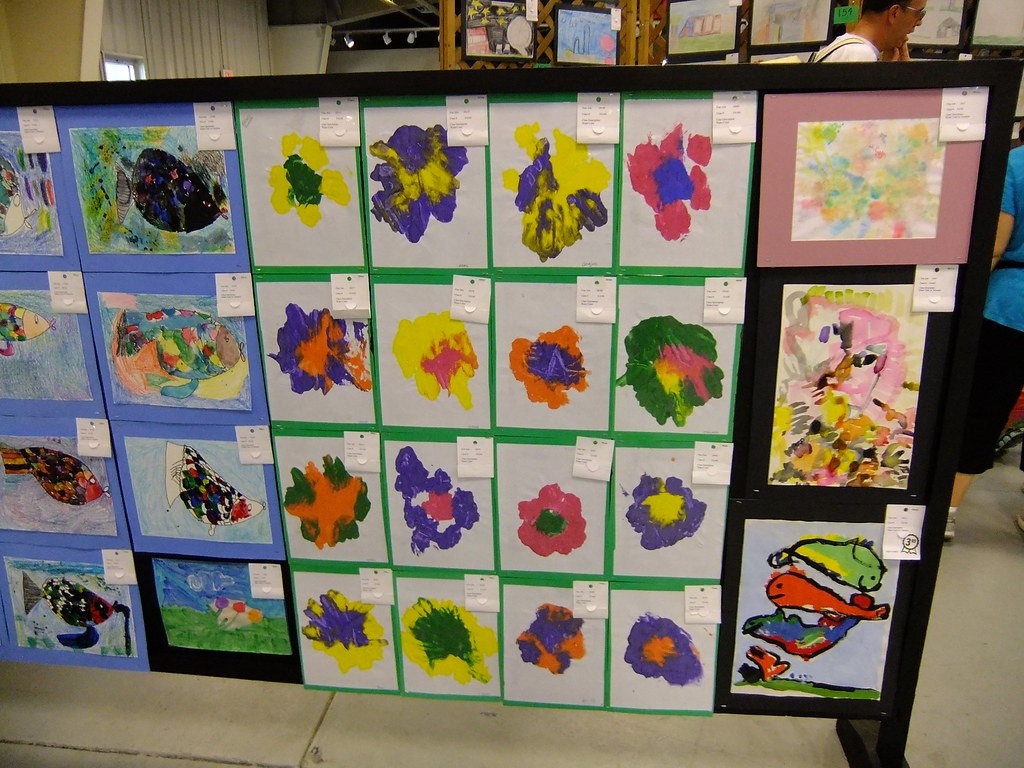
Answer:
[943,513,955,540]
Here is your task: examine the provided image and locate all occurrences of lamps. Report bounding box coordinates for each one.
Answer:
[383,32,392,45]
[407,31,417,43]
[344,33,354,48]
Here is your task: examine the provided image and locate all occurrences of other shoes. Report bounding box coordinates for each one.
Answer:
[1017,515,1024,531]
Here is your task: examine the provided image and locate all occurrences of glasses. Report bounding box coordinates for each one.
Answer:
[899,3,926,21]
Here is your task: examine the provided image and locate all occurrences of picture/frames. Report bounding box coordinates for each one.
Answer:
[666,0,1024,64]
[461,0,537,62]
[555,4,620,66]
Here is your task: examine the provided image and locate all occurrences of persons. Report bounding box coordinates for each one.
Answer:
[813,0,927,62]
[944,127,1024,541]
